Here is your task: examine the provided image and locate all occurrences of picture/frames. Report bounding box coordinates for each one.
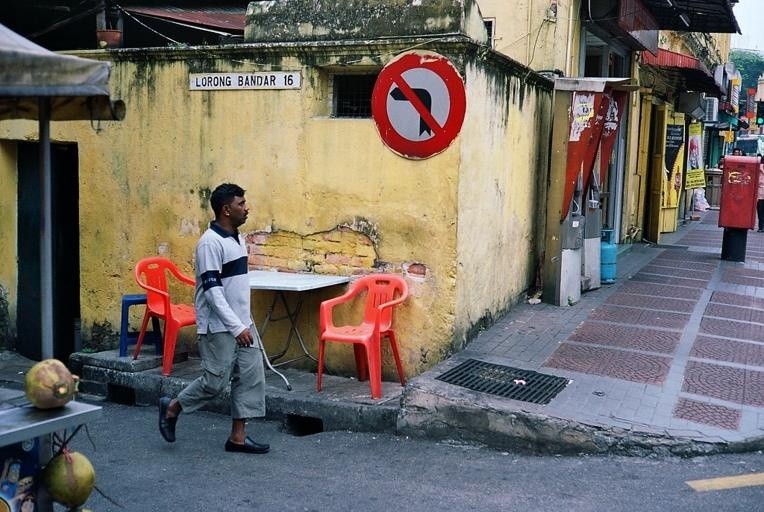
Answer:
[248,269,350,391]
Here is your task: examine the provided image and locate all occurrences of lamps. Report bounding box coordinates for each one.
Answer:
[133,257,197,377]
[316,272,410,400]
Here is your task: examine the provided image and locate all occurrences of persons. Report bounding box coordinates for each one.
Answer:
[717,146,764,233]
[154,182,271,455]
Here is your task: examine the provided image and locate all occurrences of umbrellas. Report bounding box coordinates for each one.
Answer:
[226,436,270,454]
[158,396,178,444]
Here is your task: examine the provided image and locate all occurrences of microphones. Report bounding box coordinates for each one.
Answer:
[119,294,163,357]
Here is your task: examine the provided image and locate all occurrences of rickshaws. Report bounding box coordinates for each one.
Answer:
[45,451,95,507]
[24,358,75,410]
